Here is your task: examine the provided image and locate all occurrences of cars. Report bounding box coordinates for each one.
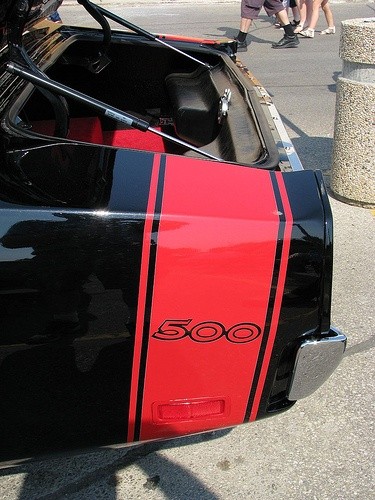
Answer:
[0,0,347,460]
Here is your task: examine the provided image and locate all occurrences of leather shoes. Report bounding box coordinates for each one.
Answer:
[274,33,299,49]
[233,38,247,52]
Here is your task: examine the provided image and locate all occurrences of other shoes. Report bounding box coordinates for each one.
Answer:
[55,19,62,23]
[321,26,335,34]
[280,26,285,31]
[299,27,315,37]
[275,22,280,27]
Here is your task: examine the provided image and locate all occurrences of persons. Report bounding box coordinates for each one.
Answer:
[235,0,300,52]
[275,0,335,37]
[274,240,325,302]
[0,213,156,344]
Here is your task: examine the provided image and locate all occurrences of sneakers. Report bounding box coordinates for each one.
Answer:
[294,25,303,33]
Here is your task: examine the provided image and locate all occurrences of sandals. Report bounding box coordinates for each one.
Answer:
[290,20,300,26]
